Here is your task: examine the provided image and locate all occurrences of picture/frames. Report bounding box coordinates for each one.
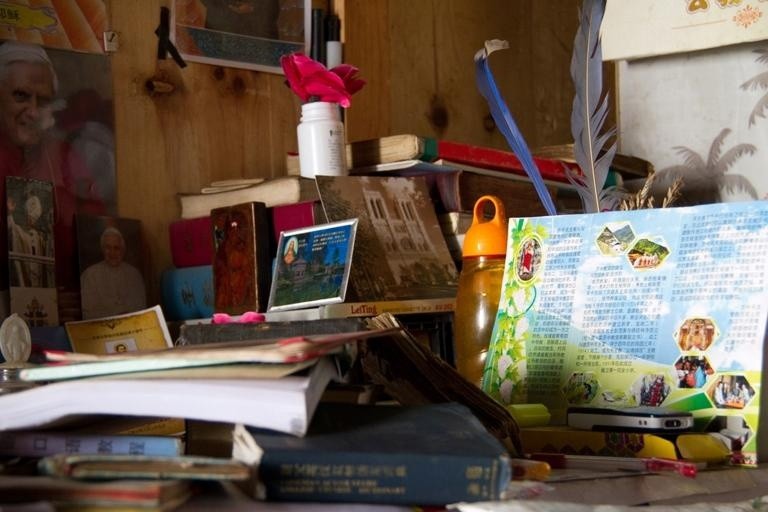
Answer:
[157,0,323,88]
[267,217,359,313]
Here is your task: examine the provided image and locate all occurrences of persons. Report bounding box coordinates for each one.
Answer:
[713,382,750,408]
[675,360,713,388]
[0,40,113,252]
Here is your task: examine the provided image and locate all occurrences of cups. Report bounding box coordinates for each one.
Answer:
[452,194,509,391]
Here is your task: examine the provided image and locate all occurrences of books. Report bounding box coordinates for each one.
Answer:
[0,134,652,512]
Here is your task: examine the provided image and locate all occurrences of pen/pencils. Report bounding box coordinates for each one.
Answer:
[524,451,698,478]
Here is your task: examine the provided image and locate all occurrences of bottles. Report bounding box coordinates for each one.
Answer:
[296,102,349,180]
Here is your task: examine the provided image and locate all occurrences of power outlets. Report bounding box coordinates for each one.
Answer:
[103,29,120,53]
